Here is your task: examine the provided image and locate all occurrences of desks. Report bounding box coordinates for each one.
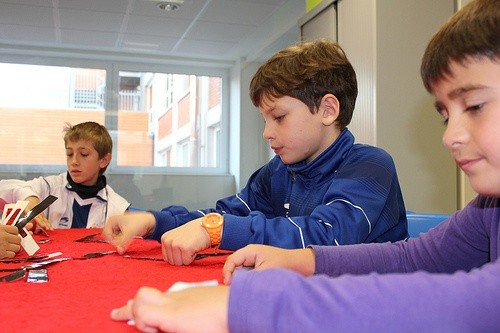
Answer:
[0,229,233,333]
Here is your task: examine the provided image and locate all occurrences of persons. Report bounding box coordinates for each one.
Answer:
[111,0,500,333]
[0,223,33,260]
[102,39,409,265]
[12,121,133,230]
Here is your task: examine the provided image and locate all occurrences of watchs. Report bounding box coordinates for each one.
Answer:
[202,213,224,246]
